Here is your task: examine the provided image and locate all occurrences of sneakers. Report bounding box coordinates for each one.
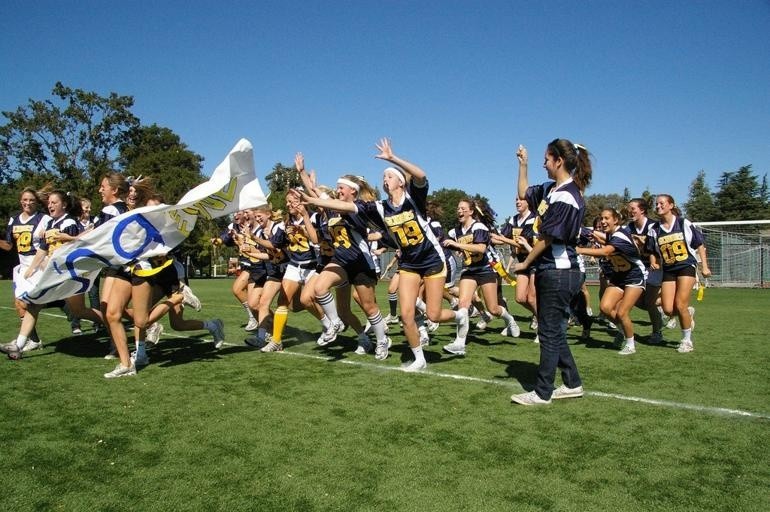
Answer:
[0,340,43,359]
[552,384,583,399]
[317,318,346,346]
[618,345,636,355]
[419,312,439,346]
[102,322,164,380]
[676,340,693,352]
[354,313,398,359]
[210,317,225,349]
[404,358,426,371]
[530,315,539,330]
[451,297,520,337]
[511,390,552,405]
[687,307,696,332]
[666,321,677,329]
[443,342,466,355]
[244,316,282,353]
[179,281,203,312]
